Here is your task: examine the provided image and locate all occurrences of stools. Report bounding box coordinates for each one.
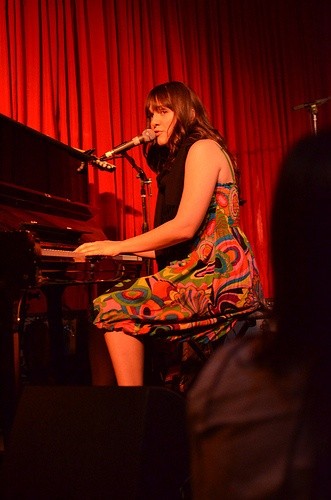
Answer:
[187,320,257,361]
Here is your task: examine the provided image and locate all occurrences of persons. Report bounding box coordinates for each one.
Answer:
[73,80,264,386]
[166,124,331,500]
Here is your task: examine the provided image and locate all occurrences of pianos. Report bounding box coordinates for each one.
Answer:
[0,113,144,394]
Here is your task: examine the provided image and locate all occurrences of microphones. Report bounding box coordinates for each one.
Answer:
[76,149,116,173]
[100,129,155,161]
